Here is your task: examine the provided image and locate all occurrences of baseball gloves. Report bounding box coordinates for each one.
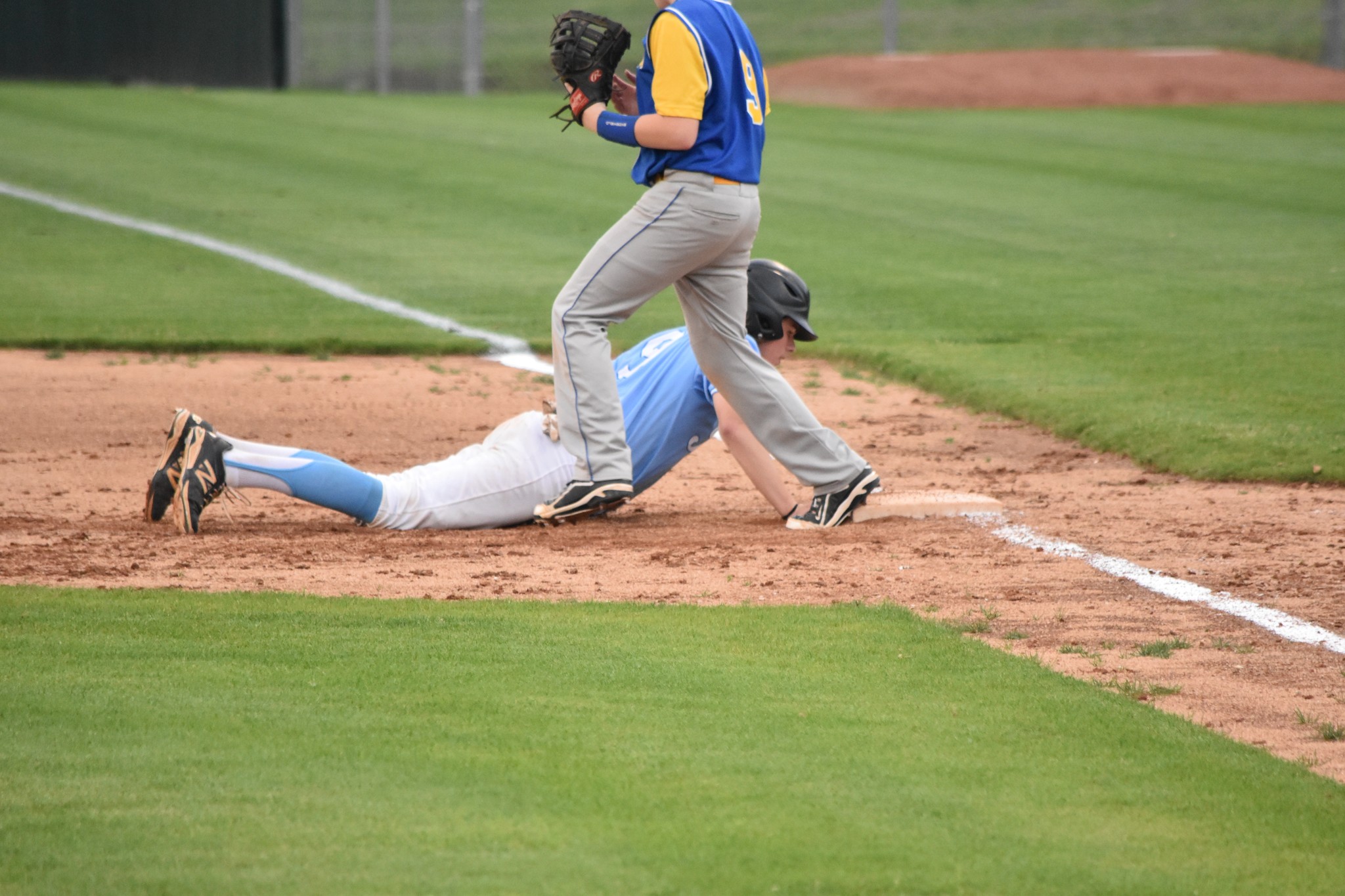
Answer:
[551,9,632,128]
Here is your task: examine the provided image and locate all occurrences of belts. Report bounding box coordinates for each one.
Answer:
[654,173,739,186]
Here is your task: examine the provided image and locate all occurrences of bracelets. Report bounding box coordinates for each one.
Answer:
[781,502,798,520]
[597,109,642,148]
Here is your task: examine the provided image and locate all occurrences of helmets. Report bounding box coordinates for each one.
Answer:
[746,260,819,343]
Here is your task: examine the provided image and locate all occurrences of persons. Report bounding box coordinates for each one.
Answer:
[533,0,880,529]
[144,257,810,534]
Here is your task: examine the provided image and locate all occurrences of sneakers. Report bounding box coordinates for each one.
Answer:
[533,478,634,526]
[143,409,213,522]
[786,463,880,531]
[174,426,250,535]
